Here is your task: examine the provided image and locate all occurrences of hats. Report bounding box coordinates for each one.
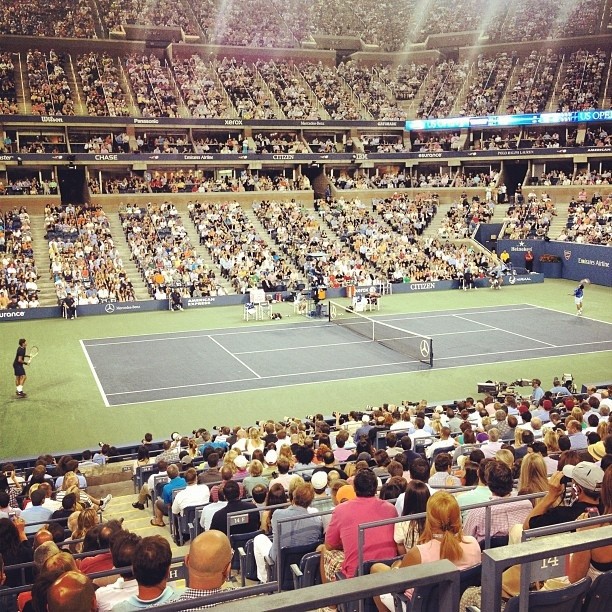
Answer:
[309,471,330,490]
[361,415,371,422]
[435,405,444,413]
[263,449,280,465]
[561,459,605,490]
[563,397,574,407]
[233,453,249,468]
[572,405,583,414]
[398,406,408,414]
[588,442,606,458]
[180,455,194,466]
[518,405,530,414]
[542,400,552,408]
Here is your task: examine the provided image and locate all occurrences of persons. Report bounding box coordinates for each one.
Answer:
[13,339,31,396]
[568,284,584,315]
[62,293,77,319]
[251,199,385,288]
[418,239,510,290]
[497,183,556,240]
[1,51,610,122]
[118,200,230,300]
[1,0,612,46]
[318,196,457,284]
[131,376,611,612]
[2,206,40,310]
[0,130,612,196]
[44,202,136,305]
[371,190,439,245]
[438,191,495,240]
[188,200,297,293]
[525,250,533,273]
[558,189,612,246]
[170,289,185,311]
[1,443,186,610]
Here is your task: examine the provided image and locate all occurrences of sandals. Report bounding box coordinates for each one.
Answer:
[150,517,166,527]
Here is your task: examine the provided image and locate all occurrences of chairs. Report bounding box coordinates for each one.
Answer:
[365,294,379,312]
[61,302,77,319]
[0,448,135,612]
[259,301,272,320]
[380,281,393,295]
[459,278,472,290]
[244,304,258,321]
[293,300,308,315]
[169,293,182,310]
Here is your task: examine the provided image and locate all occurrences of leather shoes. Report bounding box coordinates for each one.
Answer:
[131,500,146,510]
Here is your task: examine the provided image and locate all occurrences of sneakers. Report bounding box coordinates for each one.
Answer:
[99,493,113,507]
[16,391,27,398]
[576,309,583,317]
